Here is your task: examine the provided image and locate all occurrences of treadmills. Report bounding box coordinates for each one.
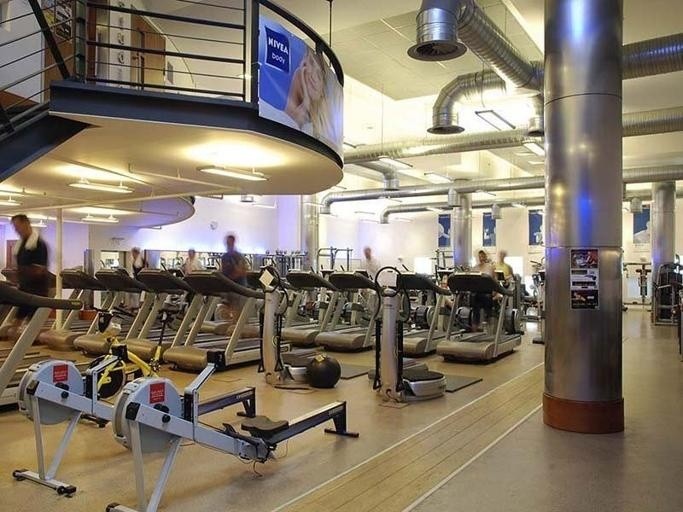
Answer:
[0,265,521,406]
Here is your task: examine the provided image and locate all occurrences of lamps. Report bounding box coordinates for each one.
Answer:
[0,110,546,225]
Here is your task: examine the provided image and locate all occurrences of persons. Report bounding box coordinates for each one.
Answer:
[215,234,248,327]
[282,43,343,141]
[180,249,206,305]
[491,249,514,285]
[358,245,380,281]
[0,213,49,348]
[583,250,597,265]
[468,248,498,334]
[125,246,149,311]
